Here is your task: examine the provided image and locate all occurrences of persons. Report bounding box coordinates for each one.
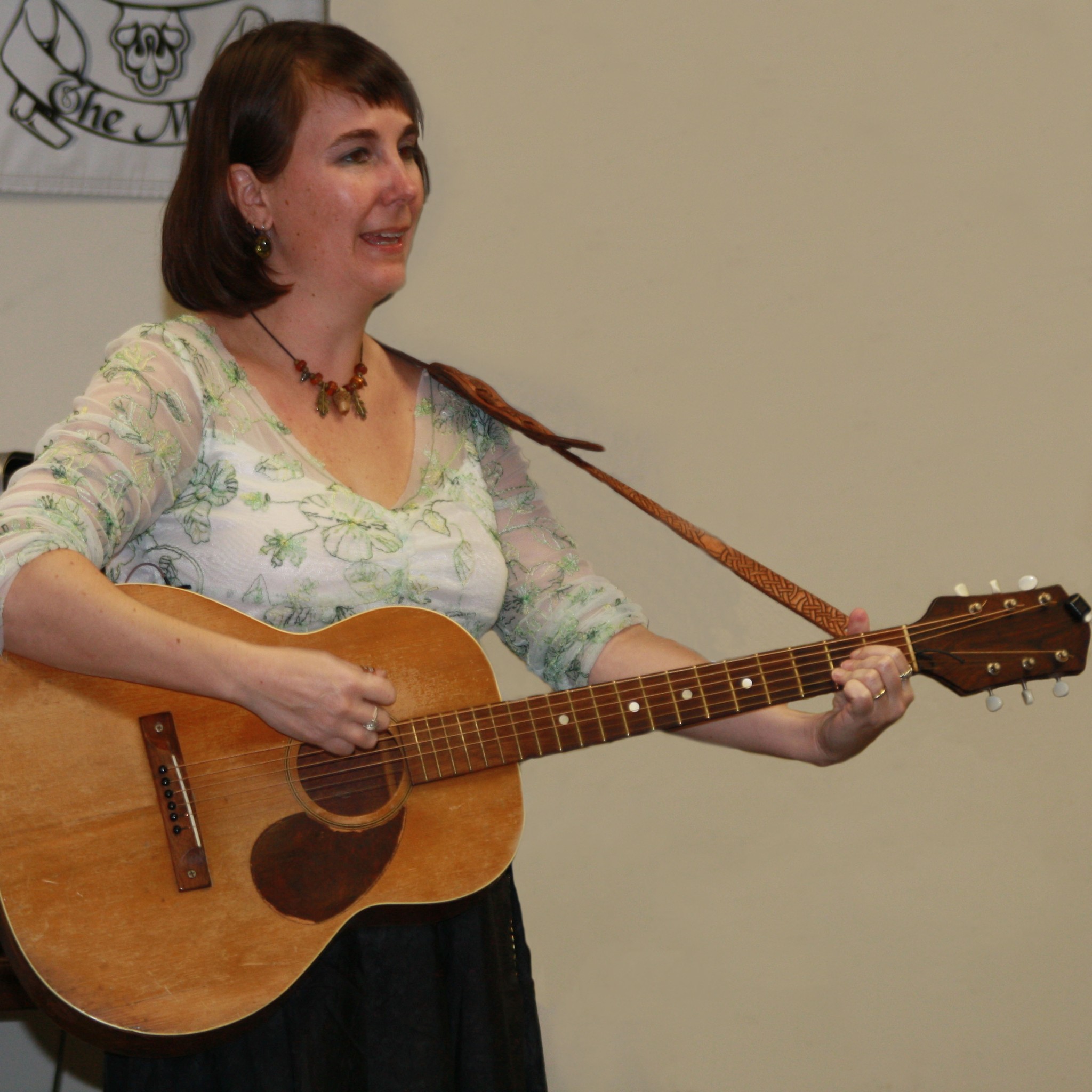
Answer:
[0,19,915,1092]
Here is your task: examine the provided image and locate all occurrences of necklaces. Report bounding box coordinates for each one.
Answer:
[249,310,369,418]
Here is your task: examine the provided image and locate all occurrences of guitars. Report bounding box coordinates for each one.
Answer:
[0,573,1092,1040]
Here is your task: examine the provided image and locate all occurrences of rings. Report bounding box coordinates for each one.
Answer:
[900,664,913,681]
[874,689,886,699]
[363,704,378,731]
